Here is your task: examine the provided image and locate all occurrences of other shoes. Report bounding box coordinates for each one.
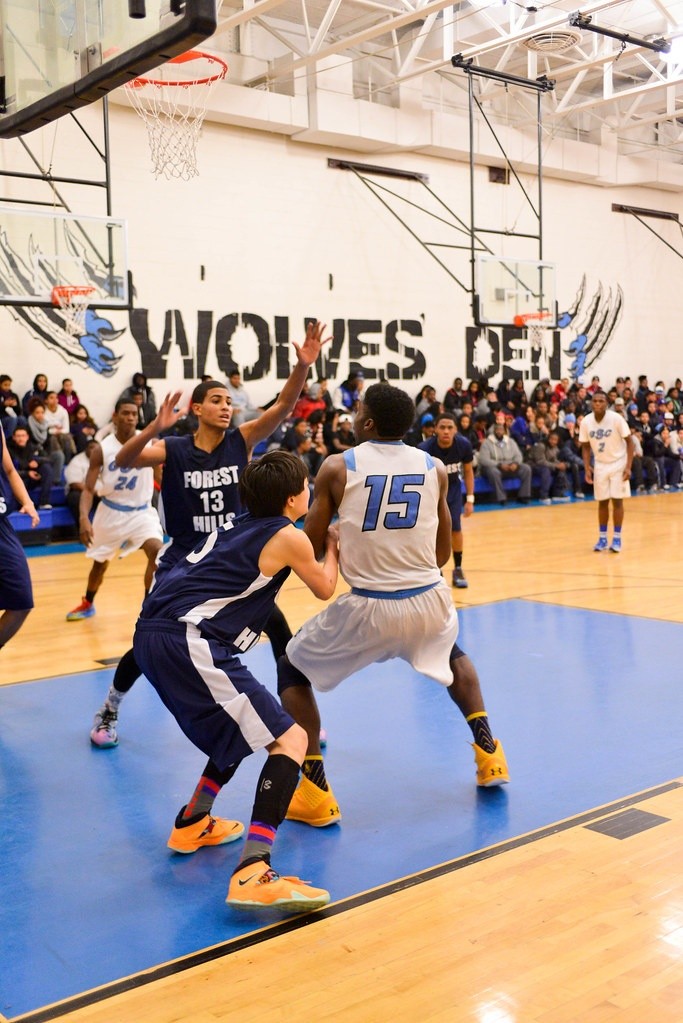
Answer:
[540,493,571,504]
[39,503,52,510]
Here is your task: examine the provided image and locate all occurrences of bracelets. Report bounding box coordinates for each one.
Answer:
[467,494,475,503]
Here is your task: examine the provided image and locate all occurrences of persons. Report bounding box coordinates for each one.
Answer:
[133,447,338,912]
[90,320,334,748]
[0,373,683,650]
[277,383,510,827]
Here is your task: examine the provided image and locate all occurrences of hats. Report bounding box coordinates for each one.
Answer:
[655,422,663,431]
[664,412,674,419]
[615,397,625,405]
[421,413,433,426]
[562,398,572,408]
[564,413,576,423]
[338,413,353,424]
[658,397,672,404]
[655,385,664,395]
[346,370,365,382]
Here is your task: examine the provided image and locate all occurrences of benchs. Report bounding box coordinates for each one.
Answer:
[6,466,673,545]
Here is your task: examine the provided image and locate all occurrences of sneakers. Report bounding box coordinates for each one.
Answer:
[67,595,95,621]
[471,738,510,787]
[166,804,245,854]
[609,538,622,552]
[453,569,467,587]
[224,861,330,912]
[89,705,120,748]
[285,775,343,827]
[593,537,608,551]
